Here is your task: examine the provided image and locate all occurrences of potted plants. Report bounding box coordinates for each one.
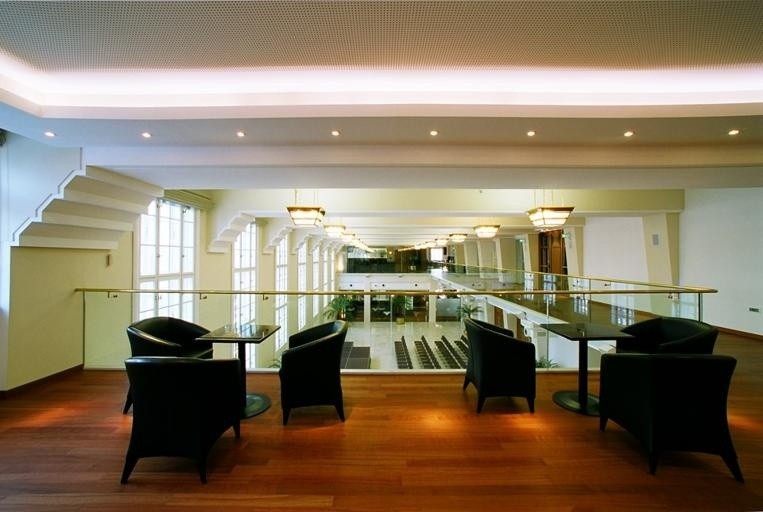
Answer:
[391,295,413,324]
[454,304,484,335]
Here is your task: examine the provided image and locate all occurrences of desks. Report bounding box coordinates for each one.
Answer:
[195,323,281,419]
[540,322,635,415]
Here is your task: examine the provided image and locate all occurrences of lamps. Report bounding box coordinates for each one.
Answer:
[285,188,325,229]
[472,215,501,240]
[450,233,467,243]
[524,187,576,234]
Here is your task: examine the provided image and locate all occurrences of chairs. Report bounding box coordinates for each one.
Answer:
[597,354,746,484]
[463,318,537,414]
[615,317,721,353]
[394,336,469,369]
[278,319,349,426]
[119,355,241,485]
[123,317,213,416]
